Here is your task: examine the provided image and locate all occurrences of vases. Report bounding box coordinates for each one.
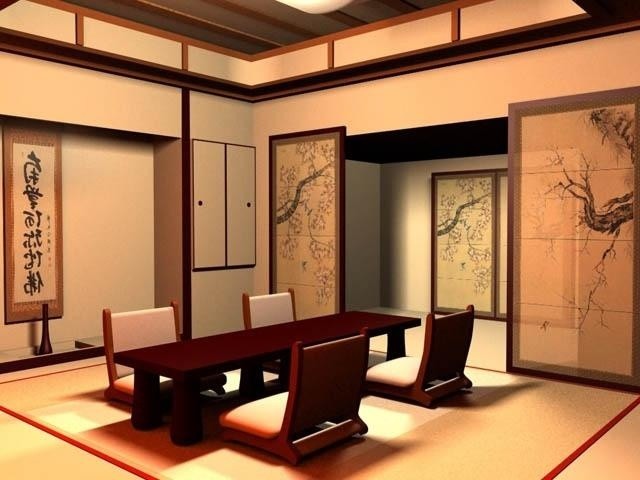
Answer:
[37,303,53,355]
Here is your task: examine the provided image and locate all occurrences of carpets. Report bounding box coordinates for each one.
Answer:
[0,350,640,480]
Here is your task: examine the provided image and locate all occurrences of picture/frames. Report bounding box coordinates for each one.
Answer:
[492,168,510,322]
[428,169,499,317]
[269,126,345,321]
[506,85,638,392]
[3,126,63,325]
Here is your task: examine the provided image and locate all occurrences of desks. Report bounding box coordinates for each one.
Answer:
[112,310,422,445]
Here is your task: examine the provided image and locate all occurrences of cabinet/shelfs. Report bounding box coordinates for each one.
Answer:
[192,139,257,271]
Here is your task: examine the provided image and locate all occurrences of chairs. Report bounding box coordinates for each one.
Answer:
[218,328,368,467]
[364,305,475,407]
[102,302,226,414]
[238,288,298,377]
